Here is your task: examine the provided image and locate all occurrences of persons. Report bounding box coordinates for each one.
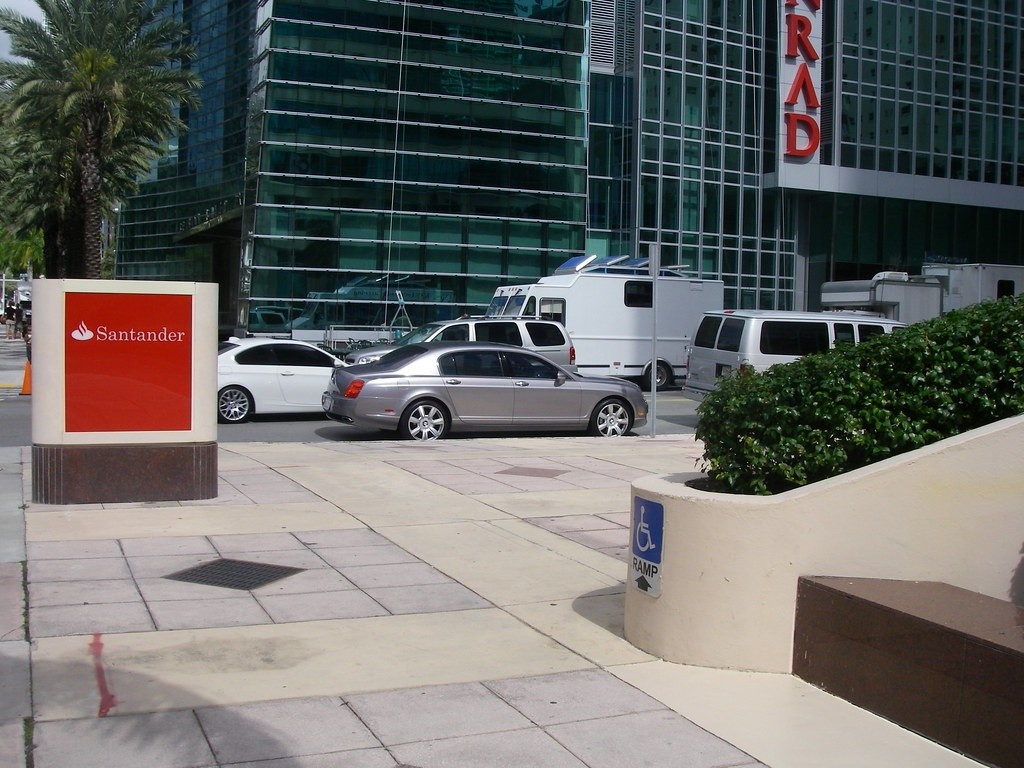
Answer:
[15,303,23,339]
[4,300,17,339]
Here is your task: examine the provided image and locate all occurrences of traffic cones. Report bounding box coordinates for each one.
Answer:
[19,359,31,395]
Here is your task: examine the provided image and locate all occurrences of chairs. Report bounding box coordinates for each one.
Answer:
[349,338,389,350]
[318,346,333,355]
[317,344,325,350]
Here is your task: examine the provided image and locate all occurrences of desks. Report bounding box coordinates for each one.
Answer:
[352,343,386,349]
[327,348,355,361]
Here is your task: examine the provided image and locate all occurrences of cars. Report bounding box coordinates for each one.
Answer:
[247,304,306,333]
[217,336,354,424]
[321,340,649,441]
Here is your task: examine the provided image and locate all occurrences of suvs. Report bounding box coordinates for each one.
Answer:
[341,312,578,374]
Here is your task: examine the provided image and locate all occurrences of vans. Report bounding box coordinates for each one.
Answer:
[283,273,461,350]
[820,261,1024,328]
[480,253,725,393]
[678,309,911,402]
